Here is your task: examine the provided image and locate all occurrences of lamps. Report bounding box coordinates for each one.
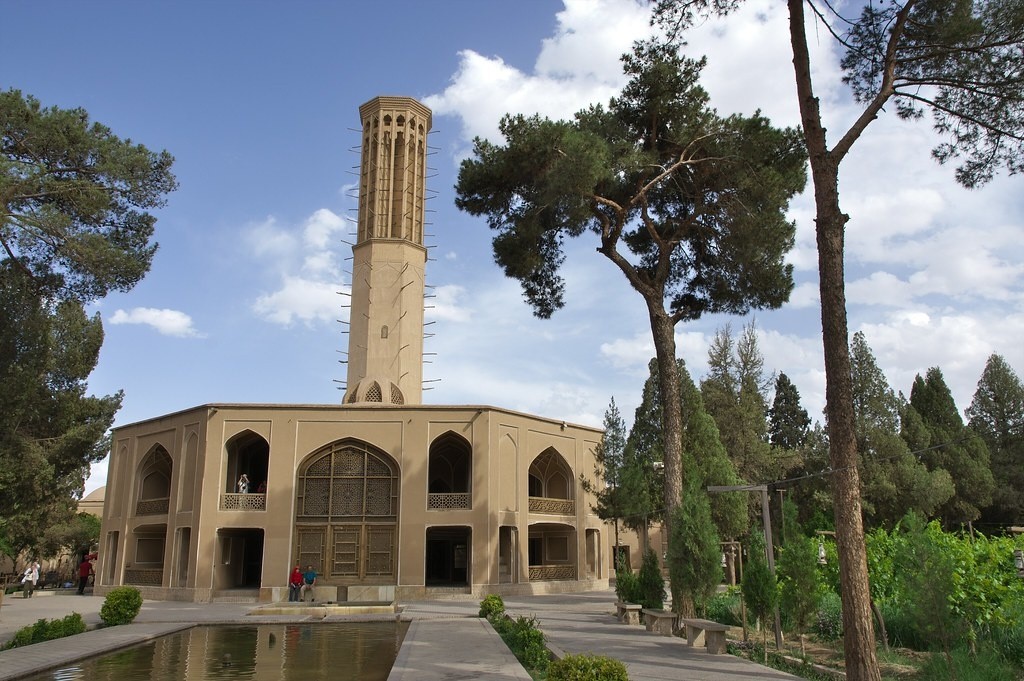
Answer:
[211,408,219,412]
[561,421,568,429]
[817,542,828,565]
[478,408,482,414]
[1014,550,1024,579]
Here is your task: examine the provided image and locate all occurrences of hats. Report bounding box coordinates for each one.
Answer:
[296,565,301,569]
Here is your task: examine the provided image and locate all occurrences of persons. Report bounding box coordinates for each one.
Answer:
[238,474,249,509]
[300,565,316,602]
[76,555,95,595]
[289,565,302,602]
[21,562,40,598]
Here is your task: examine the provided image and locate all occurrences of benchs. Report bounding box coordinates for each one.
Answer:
[272,585,338,602]
[682,618,730,654]
[347,585,402,602]
[642,609,678,636]
[614,602,642,625]
